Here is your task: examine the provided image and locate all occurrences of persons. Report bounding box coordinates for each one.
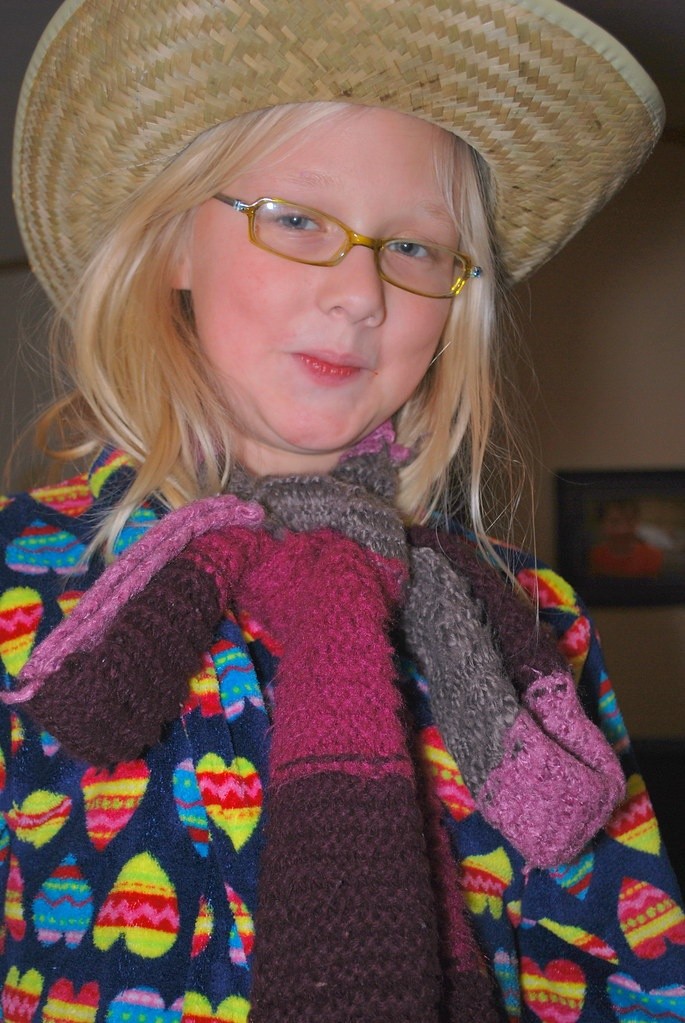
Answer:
[0,0,685,1023]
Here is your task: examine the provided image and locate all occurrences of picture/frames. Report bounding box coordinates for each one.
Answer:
[554,468,685,609]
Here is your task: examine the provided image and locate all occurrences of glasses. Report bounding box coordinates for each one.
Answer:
[212,192,484,300]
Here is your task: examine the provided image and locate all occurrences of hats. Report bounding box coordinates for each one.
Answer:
[13,0,667,346]
